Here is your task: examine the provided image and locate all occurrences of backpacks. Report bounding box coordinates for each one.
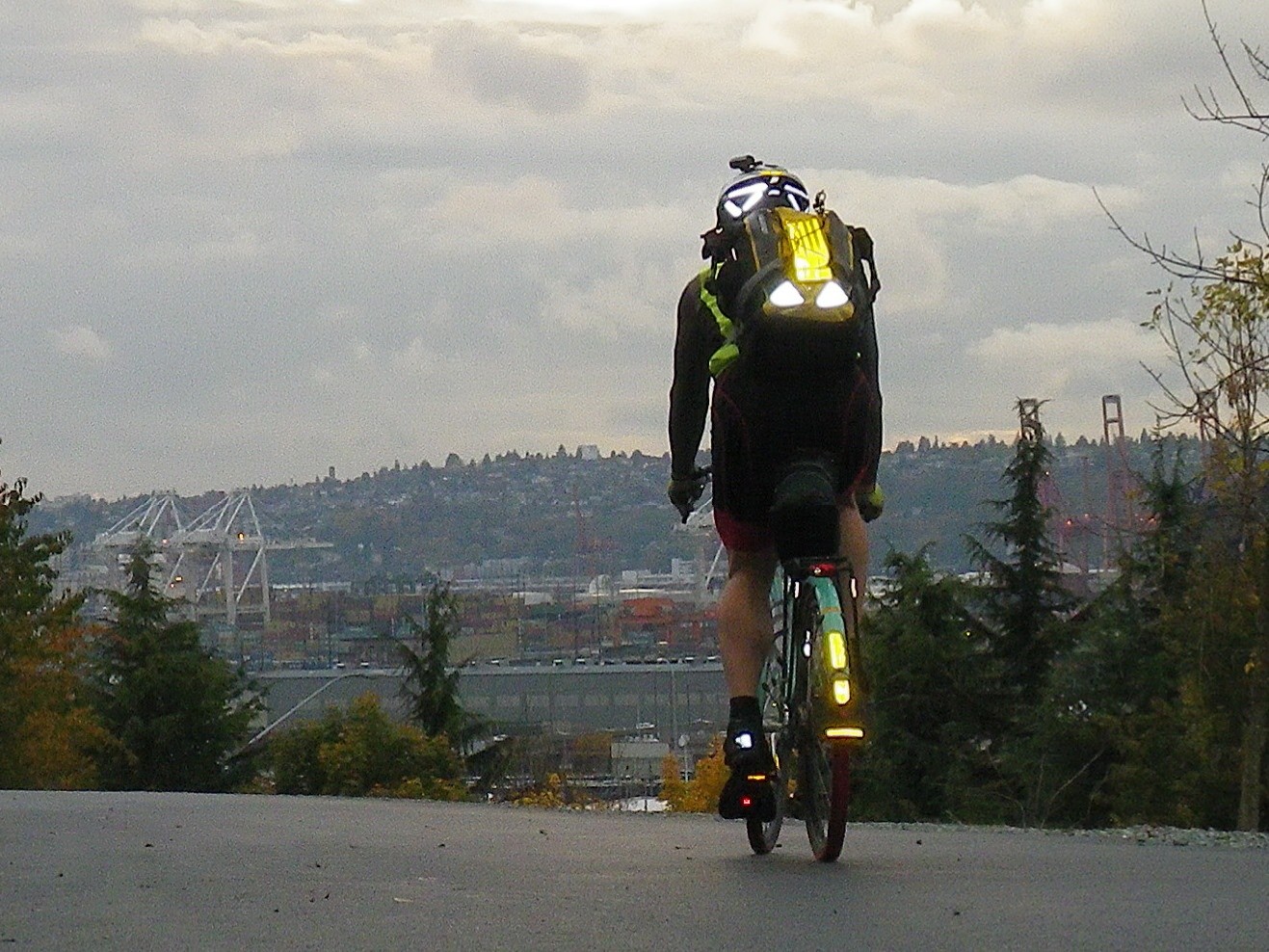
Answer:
[700,208,881,337]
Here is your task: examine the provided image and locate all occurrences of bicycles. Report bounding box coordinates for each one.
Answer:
[680,462,864,862]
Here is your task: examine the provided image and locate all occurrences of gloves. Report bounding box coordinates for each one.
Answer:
[855,483,885,523]
[668,464,703,516]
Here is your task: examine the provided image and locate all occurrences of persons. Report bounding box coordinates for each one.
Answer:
[666,153,888,822]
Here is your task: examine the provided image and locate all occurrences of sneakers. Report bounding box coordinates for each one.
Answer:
[723,719,767,766]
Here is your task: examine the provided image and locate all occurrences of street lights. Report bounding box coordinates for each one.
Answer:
[656,657,678,746]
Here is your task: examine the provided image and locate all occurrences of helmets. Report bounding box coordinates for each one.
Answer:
[717,165,810,222]
